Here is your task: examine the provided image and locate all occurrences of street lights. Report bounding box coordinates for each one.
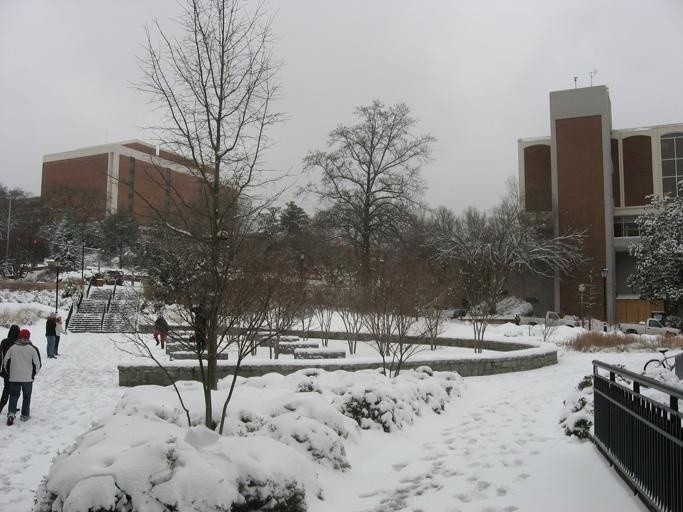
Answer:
[300,251,305,283]
[79,239,87,285]
[51,256,64,315]
[578,283,587,328]
[601,264,609,332]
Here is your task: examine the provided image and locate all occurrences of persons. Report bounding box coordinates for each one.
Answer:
[152,311,168,349]
[45,312,56,358]
[0,325,20,415]
[194,308,206,352]
[54,314,62,355]
[2,330,41,425]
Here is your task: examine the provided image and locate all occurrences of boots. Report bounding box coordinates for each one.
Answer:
[154,335,160,346]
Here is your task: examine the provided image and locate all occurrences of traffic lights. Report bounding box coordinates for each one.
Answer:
[31,239,38,245]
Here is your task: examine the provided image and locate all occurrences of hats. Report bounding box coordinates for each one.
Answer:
[48,311,61,320]
[19,329,30,339]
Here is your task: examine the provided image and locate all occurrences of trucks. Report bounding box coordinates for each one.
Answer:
[518,311,575,328]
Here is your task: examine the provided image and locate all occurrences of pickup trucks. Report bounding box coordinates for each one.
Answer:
[103,270,124,285]
[618,318,682,338]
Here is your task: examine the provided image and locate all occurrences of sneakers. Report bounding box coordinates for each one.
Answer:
[6,413,15,426]
[160,344,164,349]
[47,353,60,360]
[19,414,32,422]
